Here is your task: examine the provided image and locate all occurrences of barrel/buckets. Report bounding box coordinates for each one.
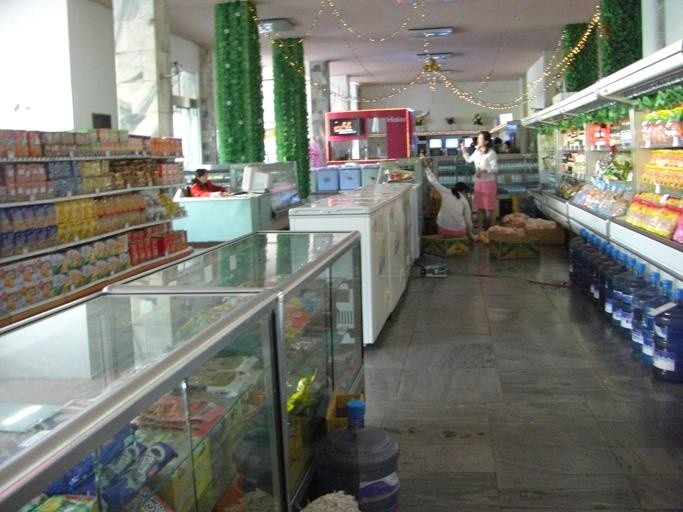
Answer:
[326,397,400,511]
[567,225,683,383]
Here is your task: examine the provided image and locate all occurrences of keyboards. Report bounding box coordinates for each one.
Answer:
[230,191,247,195]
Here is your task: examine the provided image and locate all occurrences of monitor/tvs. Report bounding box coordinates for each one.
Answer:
[242,166,270,193]
[445,138,459,148]
[429,138,443,149]
[464,138,473,148]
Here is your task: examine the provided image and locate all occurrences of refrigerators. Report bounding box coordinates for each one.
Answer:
[288,182,421,346]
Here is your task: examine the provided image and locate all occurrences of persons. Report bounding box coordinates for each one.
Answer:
[458,137,512,154]
[420,153,478,242]
[190,169,230,197]
[460,131,497,225]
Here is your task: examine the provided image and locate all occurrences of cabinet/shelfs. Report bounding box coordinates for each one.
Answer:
[2,235,359,512]
[0,155,182,326]
[530,92,683,265]
